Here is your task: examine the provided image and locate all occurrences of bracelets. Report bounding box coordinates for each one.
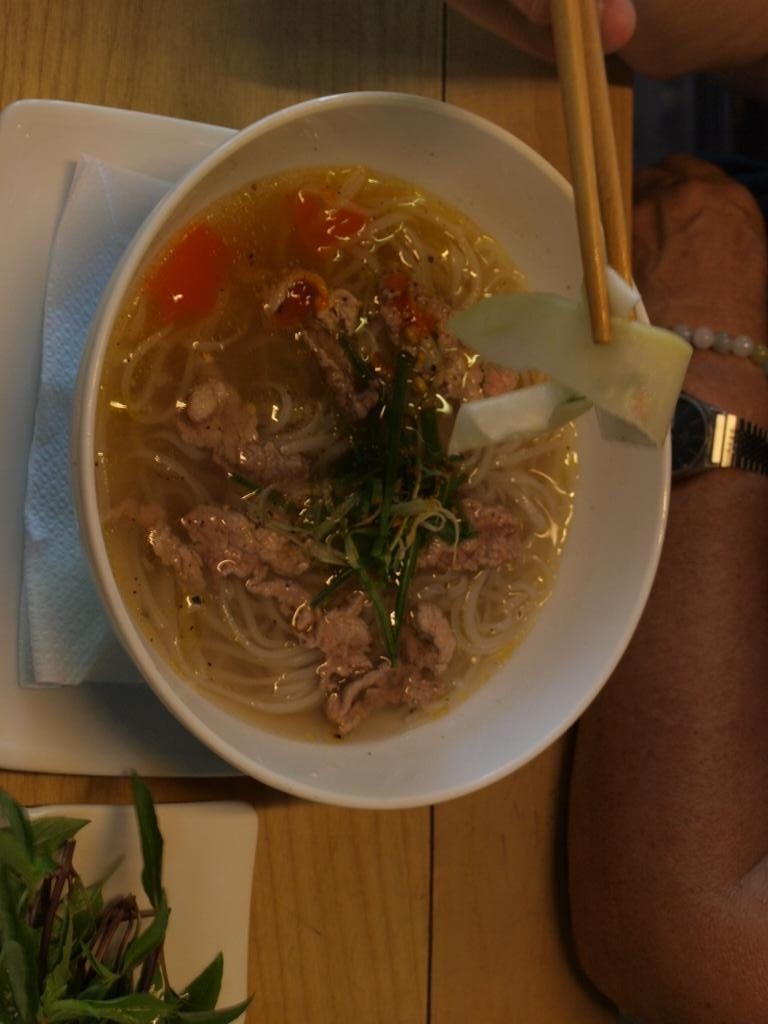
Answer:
[241,0,273,145]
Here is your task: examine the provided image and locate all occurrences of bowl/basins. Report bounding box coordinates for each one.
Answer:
[68,128,606,929]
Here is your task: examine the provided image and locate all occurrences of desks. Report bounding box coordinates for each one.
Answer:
[1,179,768,1023]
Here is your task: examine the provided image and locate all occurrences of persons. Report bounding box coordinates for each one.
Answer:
[0,0,768,432]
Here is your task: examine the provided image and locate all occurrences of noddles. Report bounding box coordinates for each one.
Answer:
[126,256,557,892]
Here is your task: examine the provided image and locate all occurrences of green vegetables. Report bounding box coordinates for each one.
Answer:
[252,387,501,716]
[578,686,768,1024]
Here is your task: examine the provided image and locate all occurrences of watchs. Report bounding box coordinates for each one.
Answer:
[293,0,365,133]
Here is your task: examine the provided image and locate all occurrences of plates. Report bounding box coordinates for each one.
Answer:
[603,679,768,1024]
[73,701,583,1024]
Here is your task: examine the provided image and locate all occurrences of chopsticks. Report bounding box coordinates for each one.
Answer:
[0,173,259,290]
